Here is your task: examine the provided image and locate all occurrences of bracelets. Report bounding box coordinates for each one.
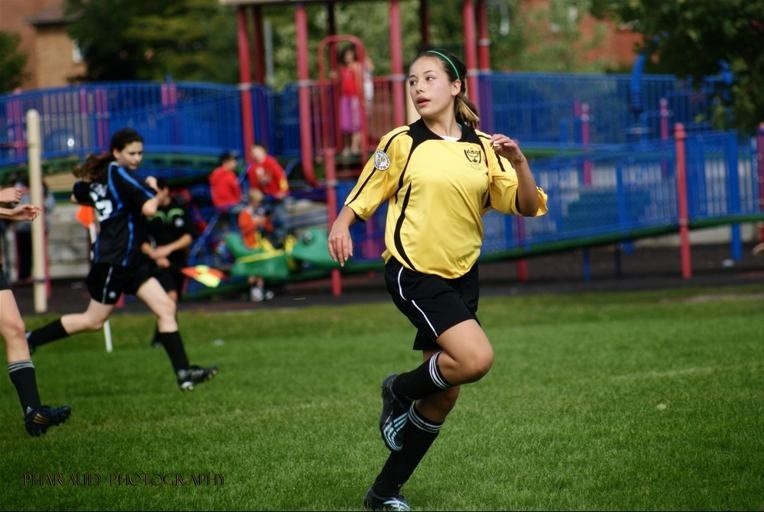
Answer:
[509,152,525,168]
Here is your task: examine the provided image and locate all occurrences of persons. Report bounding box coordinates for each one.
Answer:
[1,46,376,435]
[327,48,551,510]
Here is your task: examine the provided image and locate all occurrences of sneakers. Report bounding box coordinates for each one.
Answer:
[23,405,71,436]
[362,494,411,511]
[378,374,409,452]
[176,365,217,390]
